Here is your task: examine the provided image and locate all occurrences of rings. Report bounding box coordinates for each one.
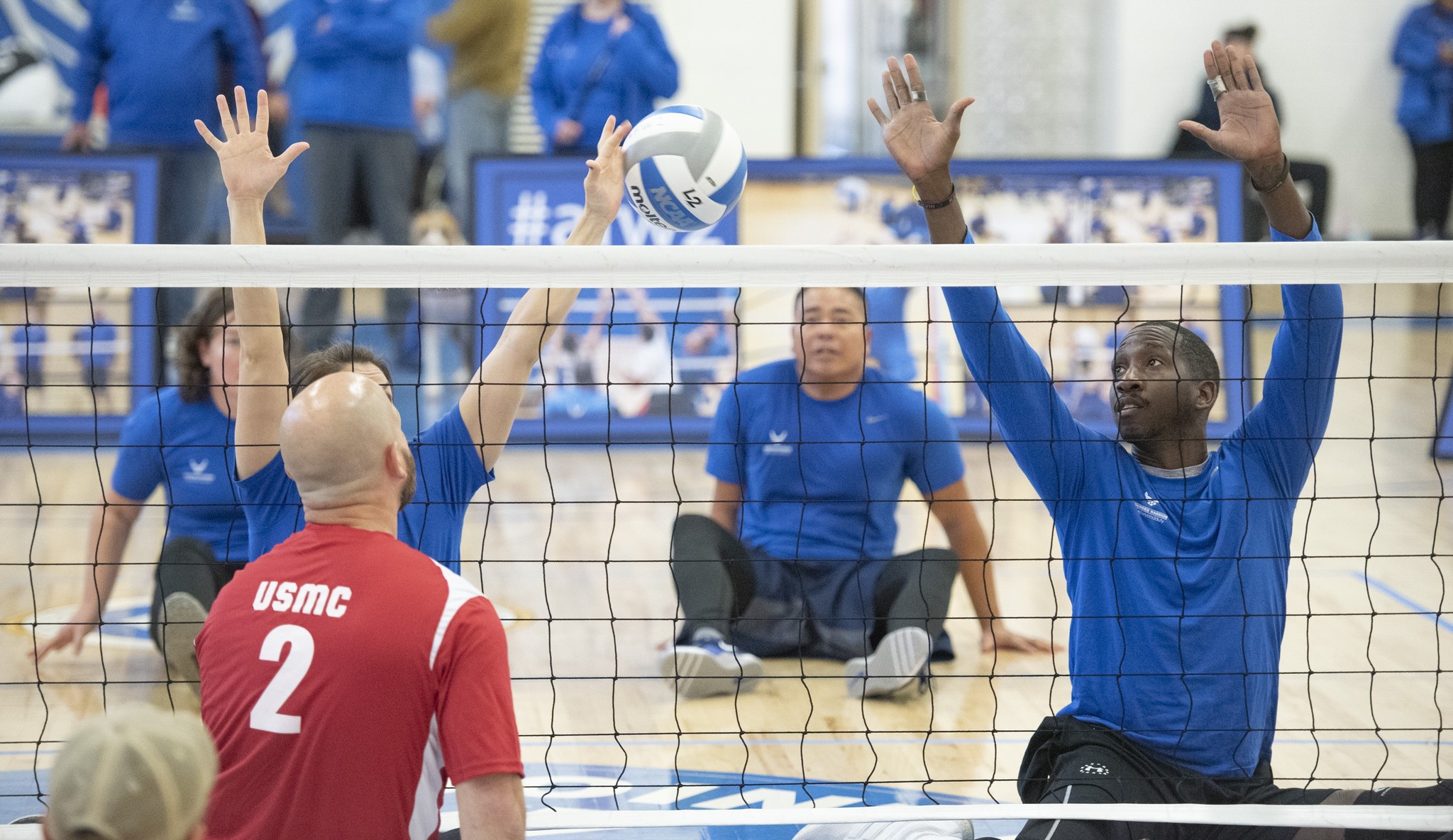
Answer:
[911,90,928,101]
[1206,75,1228,102]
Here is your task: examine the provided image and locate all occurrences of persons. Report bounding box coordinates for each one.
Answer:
[525,0,683,160]
[60,0,529,373]
[654,290,1065,703]
[862,39,1453,840]
[21,83,634,840]
[1167,20,1285,158]
[1388,0,1452,241]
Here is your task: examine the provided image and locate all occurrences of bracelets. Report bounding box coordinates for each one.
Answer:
[912,183,956,210]
[1250,152,1291,194]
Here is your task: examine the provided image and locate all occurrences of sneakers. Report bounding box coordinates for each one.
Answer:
[844,626,933,697]
[792,819,974,840]
[660,639,762,697]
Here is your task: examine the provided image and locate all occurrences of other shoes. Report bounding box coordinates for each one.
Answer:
[158,592,207,699]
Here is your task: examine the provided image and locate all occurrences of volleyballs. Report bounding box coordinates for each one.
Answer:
[620,104,747,232]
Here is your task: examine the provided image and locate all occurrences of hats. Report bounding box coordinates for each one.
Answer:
[45,703,218,840]
